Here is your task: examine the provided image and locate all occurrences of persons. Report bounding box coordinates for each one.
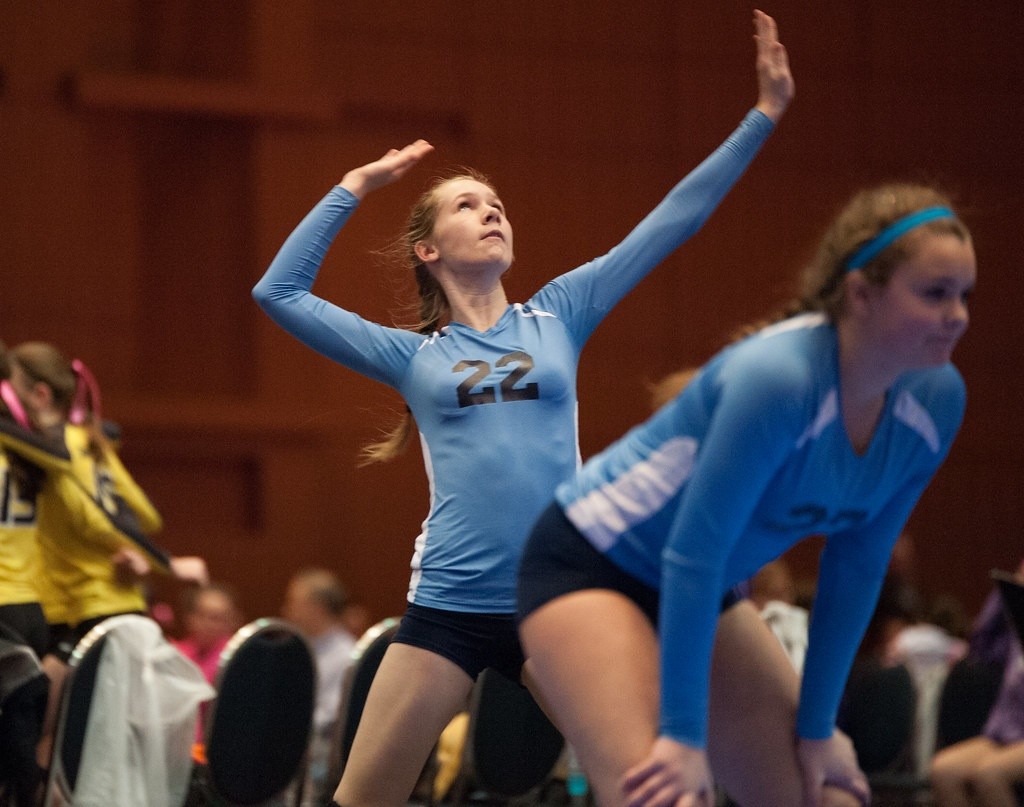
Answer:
[513,183,977,807]
[251,11,796,807]
[753,553,1024,807]
[0,345,373,807]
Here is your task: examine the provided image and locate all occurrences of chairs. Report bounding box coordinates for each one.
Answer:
[0,603,1024,807]
